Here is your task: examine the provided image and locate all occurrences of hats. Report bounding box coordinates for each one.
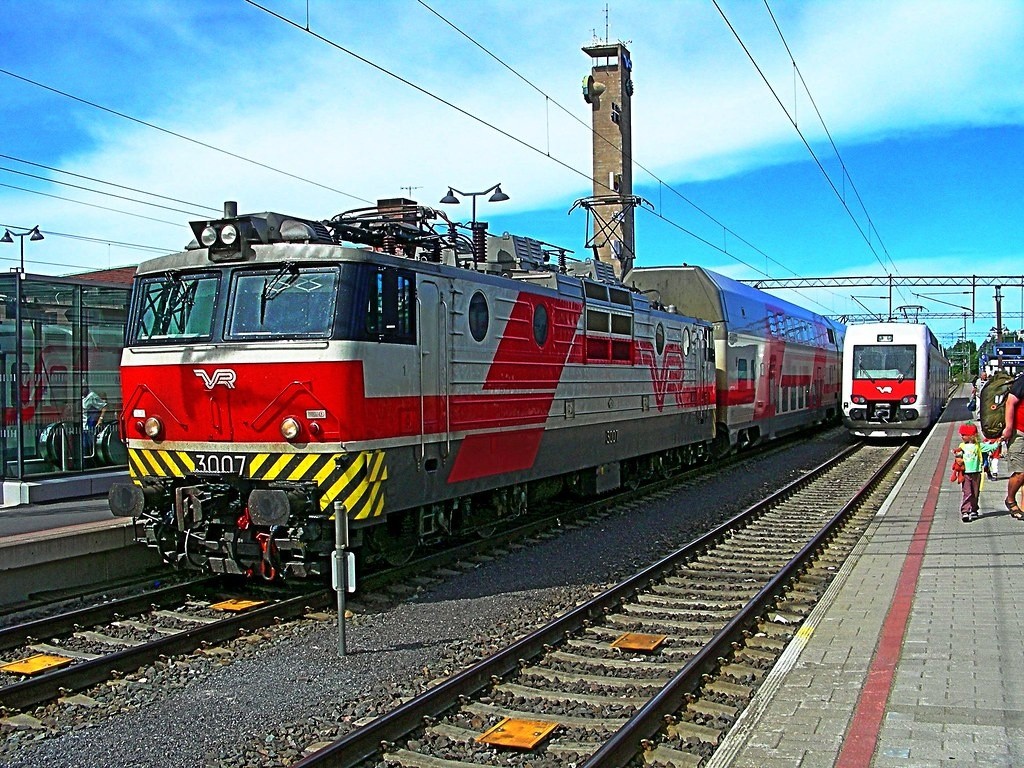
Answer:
[958,421,978,437]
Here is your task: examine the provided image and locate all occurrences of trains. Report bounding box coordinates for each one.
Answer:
[106,196,860,592]
[838,322,951,440]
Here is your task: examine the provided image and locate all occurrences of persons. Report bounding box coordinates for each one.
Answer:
[958,421,1003,522]
[73,379,108,469]
[1001,374,1024,520]
[971,369,1024,481]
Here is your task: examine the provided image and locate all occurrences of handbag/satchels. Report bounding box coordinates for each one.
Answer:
[967,397,976,411]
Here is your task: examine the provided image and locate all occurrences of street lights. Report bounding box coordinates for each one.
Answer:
[440,182,510,227]
[0,224,44,483]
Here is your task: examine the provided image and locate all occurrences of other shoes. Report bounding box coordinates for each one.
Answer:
[988,475,997,482]
[992,473,997,478]
[969,512,978,519]
[977,419,981,421]
[962,513,969,522]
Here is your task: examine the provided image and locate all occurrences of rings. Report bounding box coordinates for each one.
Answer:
[1001,437,1004,441]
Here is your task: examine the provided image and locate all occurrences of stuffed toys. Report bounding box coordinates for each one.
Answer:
[950,447,965,484]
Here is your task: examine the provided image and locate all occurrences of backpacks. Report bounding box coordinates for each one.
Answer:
[980,371,1024,439]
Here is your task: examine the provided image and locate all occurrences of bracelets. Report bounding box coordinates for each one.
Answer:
[100,417,104,420]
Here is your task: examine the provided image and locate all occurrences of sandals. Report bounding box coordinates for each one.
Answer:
[1005,496,1023,518]
[1018,511,1024,521]
